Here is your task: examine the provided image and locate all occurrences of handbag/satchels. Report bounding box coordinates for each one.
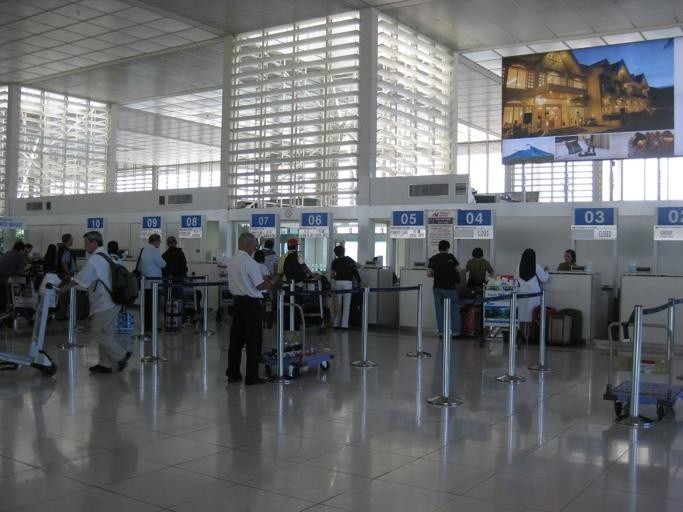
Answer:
[132,269,142,290]
[284,252,306,284]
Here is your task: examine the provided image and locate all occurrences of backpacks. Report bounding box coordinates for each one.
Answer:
[95,252,139,305]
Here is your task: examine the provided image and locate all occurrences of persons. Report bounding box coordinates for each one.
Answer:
[0,233,186,341]
[329,245,360,330]
[513,248,550,345]
[253,250,275,302]
[557,249,577,271]
[56,230,132,374]
[225,234,269,385]
[261,240,278,277]
[462,247,496,287]
[425,240,463,338]
[276,238,304,331]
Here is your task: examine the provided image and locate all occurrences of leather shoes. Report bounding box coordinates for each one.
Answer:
[246,379,267,385]
[88,365,111,373]
[118,351,131,371]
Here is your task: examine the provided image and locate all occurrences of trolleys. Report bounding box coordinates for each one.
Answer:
[261,278,333,381]
[477,281,523,349]
[176,272,204,333]
[8,271,58,334]
[603,323,682,421]
[0,274,65,377]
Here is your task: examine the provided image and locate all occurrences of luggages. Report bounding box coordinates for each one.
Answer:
[531,304,552,344]
[460,302,481,338]
[164,276,182,330]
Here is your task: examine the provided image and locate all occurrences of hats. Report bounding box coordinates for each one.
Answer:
[287,239,299,246]
